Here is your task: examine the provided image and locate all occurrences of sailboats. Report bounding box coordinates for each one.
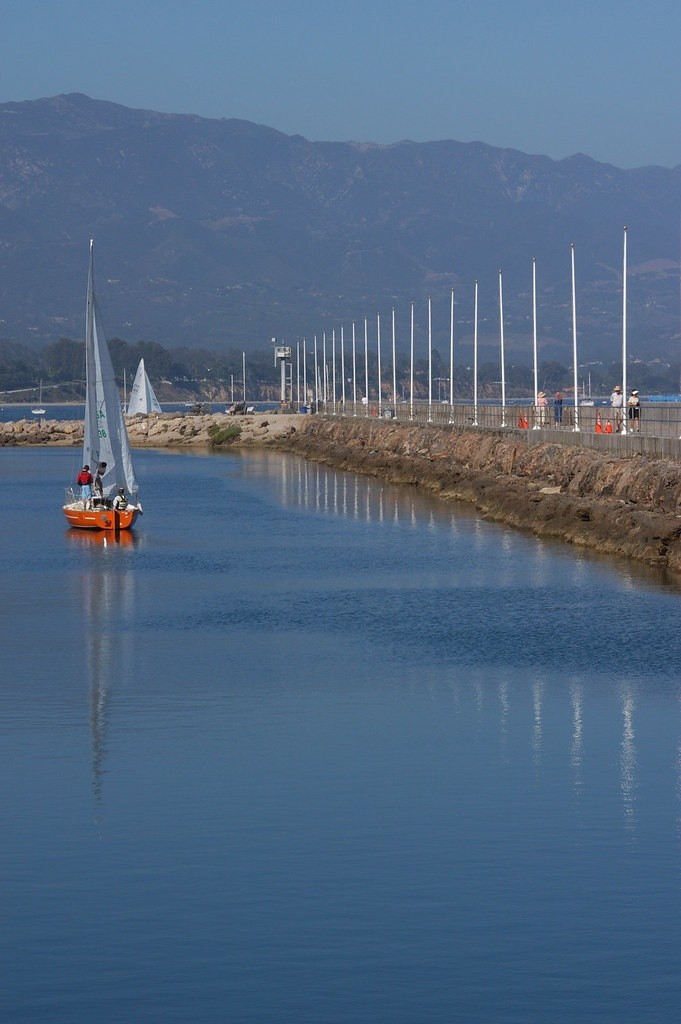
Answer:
[580,371,594,406]
[31,380,46,415]
[62,239,142,535]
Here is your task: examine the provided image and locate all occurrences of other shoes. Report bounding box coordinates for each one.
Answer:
[630,428,640,433]
[82,505,93,511]
[615,428,619,433]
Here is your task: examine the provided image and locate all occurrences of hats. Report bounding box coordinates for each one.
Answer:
[613,386,622,391]
[538,392,546,398]
[631,390,639,395]
[83,465,91,470]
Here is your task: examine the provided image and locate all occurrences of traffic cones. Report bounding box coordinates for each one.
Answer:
[522,413,529,429]
[518,415,523,427]
[602,419,613,433]
[595,412,604,433]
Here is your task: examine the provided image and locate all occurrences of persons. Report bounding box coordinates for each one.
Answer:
[337,396,345,416]
[537,391,548,428]
[553,393,563,426]
[627,389,640,432]
[112,488,129,510]
[77,465,93,511]
[610,386,623,432]
[93,462,107,498]
[362,395,367,415]
